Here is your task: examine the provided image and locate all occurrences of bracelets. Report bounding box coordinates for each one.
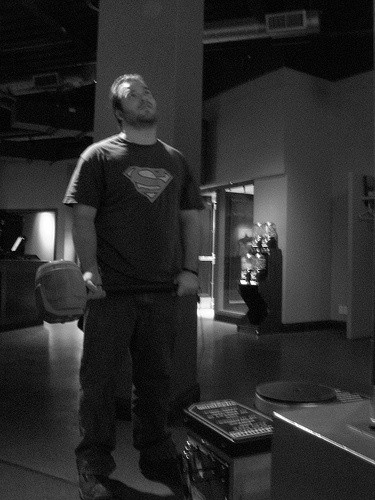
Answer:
[182,267,199,277]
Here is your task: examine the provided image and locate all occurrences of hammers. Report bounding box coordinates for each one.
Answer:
[35,258,180,324]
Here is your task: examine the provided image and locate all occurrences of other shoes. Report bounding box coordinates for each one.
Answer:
[139,444,192,495]
[76,473,114,500]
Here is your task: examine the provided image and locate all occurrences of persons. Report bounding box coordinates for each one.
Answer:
[62,74,206,500]
